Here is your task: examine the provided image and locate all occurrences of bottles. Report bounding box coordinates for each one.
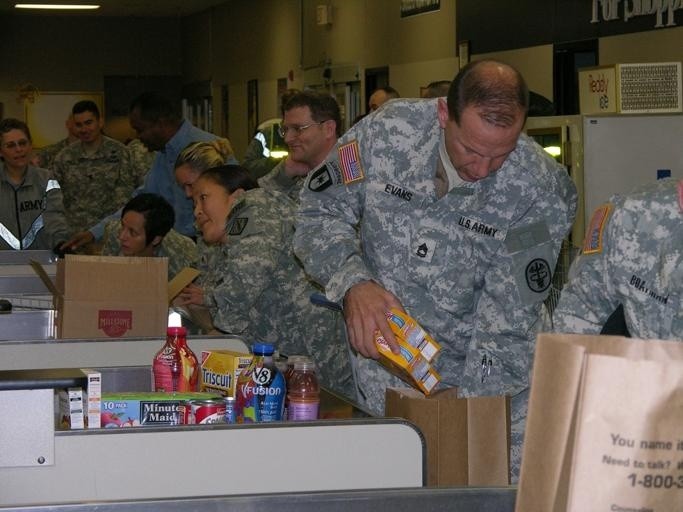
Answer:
[233,343,286,424]
[282,355,307,408]
[224,396,235,425]
[191,399,226,425]
[152,327,199,393]
[288,361,321,422]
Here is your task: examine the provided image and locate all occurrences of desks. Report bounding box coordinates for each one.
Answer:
[1,339,422,489]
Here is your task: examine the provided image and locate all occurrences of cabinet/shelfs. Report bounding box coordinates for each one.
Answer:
[303,62,388,144]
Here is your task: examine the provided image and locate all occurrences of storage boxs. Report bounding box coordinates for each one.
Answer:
[55,254,169,340]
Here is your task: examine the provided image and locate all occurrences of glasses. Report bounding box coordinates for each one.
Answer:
[277,122,320,138]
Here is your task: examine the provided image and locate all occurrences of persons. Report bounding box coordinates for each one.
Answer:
[242,88,302,178]
[369,87,400,113]
[550,174,683,345]
[172,138,234,286]
[0,117,70,250]
[177,164,357,404]
[291,59,579,483]
[100,191,196,282]
[59,85,240,251]
[258,89,342,205]
[40,110,79,167]
[45,100,134,230]
